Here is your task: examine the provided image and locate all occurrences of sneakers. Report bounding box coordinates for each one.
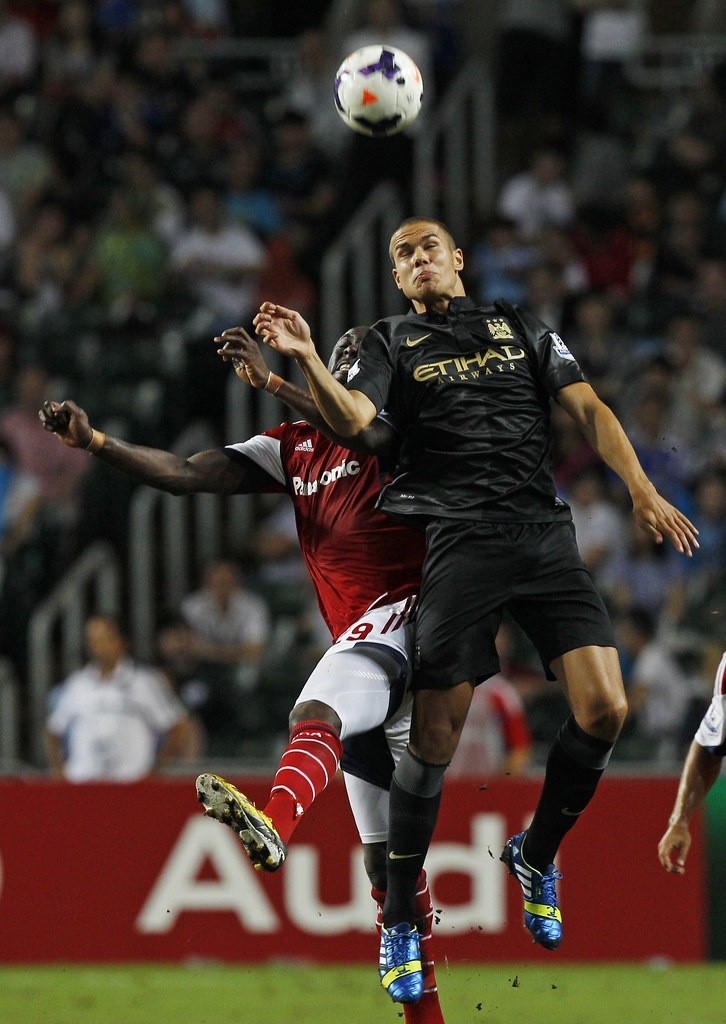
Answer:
[499,829,564,950]
[195,771,288,873]
[378,922,423,1004]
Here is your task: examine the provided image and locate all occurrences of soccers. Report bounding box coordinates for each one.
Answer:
[334,44,423,136]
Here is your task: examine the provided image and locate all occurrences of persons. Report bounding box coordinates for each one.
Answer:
[37,321,451,1024]
[2,2,726,776]
[655,646,726,876]
[250,214,700,1003]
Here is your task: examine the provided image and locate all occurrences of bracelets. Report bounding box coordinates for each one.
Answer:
[263,369,285,396]
[85,427,107,456]
